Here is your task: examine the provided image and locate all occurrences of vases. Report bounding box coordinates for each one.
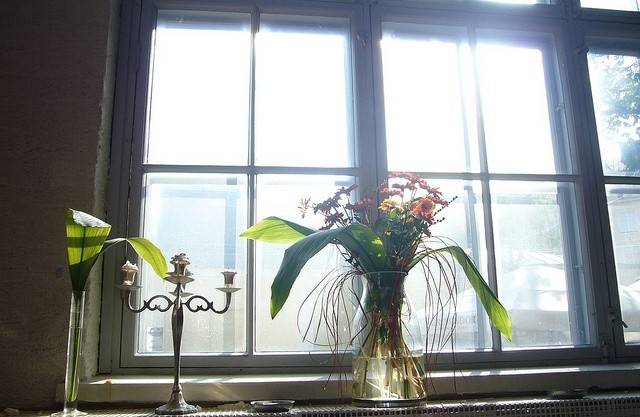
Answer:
[350,270,425,407]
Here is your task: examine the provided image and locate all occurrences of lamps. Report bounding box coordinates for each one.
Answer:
[118,252,242,412]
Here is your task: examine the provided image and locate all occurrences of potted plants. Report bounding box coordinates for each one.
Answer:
[50,207,169,417]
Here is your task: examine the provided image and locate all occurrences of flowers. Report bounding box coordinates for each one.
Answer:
[238,172,513,401]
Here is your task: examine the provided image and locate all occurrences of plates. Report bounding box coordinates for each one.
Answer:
[250,399,296,410]
